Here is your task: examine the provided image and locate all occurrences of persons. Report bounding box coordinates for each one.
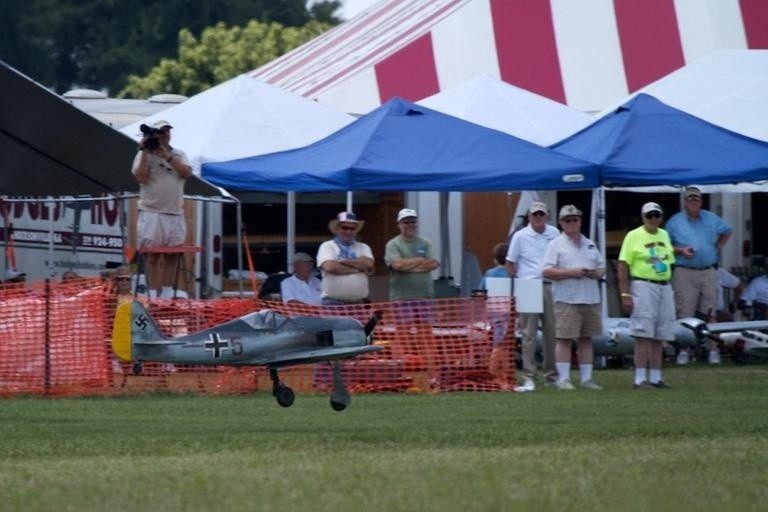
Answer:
[1,267,134,296]
[384,207,440,389]
[505,201,562,391]
[617,202,676,392]
[132,118,192,299]
[664,187,732,365]
[316,212,376,394]
[739,257,768,344]
[542,204,607,391]
[479,242,517,383]
[695,264,744,330]
[280,252,324,318]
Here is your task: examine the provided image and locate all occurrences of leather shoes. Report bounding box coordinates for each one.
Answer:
[633,380,656,390]
[650,380,673,388]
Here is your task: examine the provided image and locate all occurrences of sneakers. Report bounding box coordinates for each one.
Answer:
[544,379,559,387]
[557,381,576,391]
[706,350,722,366]
[579,381,603,390]
[513,378,536,393]
[675,351,690,365]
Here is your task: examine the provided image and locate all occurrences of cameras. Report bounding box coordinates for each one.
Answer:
[140,124,162,151]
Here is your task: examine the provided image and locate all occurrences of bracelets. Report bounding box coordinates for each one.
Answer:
[166,156,172,163]
[621,292,632,298]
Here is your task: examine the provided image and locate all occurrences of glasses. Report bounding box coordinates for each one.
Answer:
[338,225,357,231]
[686,197,701,201]
[116,278,132,282]
[644,212,661,220]
[562,218,581,223]
[401,220,416,224]
[532,211,545,217]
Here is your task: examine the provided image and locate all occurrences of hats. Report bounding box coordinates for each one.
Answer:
[396,208,418,223]
[116,265,132,279]
[529,201,549,217]
[684,187,702,199]
[327,211,365,235]
[558,204,584,221]
[152,120,174,131]
[640,201,663,215]
[294,252,313,264]
[3,267,27,282]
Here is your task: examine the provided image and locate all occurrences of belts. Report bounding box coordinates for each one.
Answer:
[675,264,717,271]
[543,279,552,285]
[631,277,668,285]
